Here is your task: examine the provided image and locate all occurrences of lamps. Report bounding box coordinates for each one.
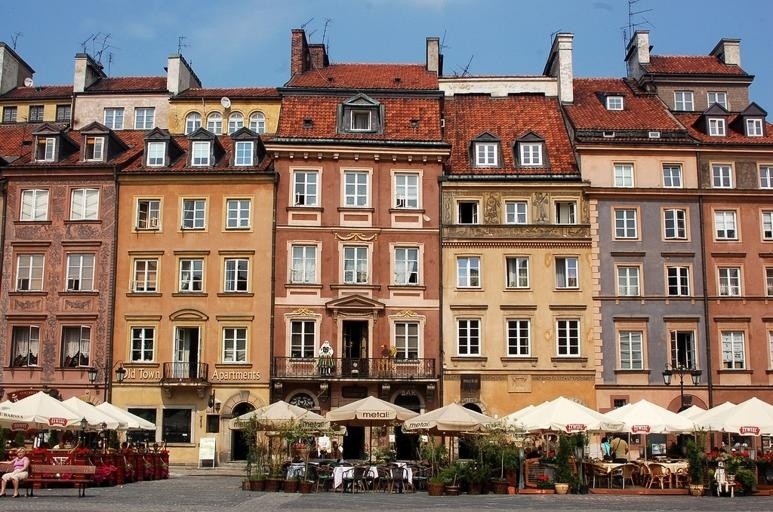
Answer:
[207,389,215,413]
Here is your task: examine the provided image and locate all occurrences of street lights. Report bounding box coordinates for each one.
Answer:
[662,360,703,412]
[100,420,107,454]
[87,358,127,404]
[79,416,88,448]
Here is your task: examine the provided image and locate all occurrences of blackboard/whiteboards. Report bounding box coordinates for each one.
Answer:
[199,438,215,460]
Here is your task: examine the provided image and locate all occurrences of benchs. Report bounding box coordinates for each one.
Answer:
[0,462,96,499]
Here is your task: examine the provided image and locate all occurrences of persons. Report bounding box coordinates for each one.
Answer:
[713,461,729,495]
[485,192,501,226]
[1,447,31,497]
[532,192,551,224]
[379,342,391,364]
[610,433,628,463]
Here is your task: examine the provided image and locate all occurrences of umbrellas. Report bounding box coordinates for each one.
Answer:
[0,391,156,448]
[401,401,495,463]
[496,395,773,460]
[265,422,349,459]
[325,395,418,464]
[228,400,326,465]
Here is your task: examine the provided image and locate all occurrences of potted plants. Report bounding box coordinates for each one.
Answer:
[415,412,592,496]
[231,408,337,494]
[684,436,757,497]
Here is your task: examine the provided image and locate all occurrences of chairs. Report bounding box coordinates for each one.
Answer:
[303,458,432,496]
[581,456,689,489]
